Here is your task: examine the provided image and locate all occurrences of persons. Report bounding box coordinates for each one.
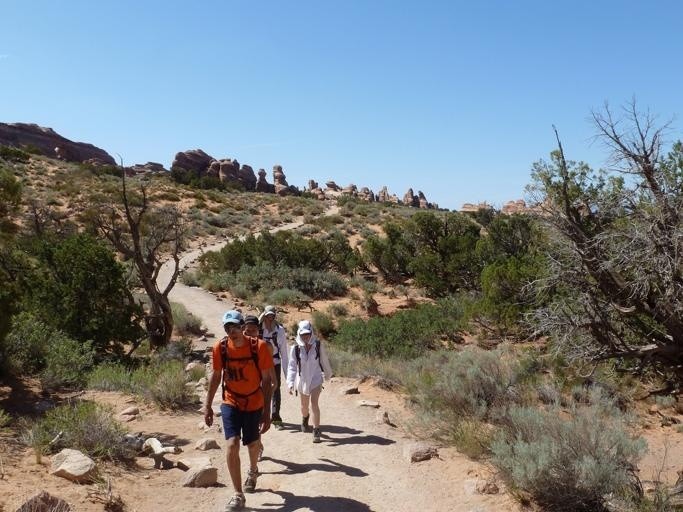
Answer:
[245,314,278,462]
[285,319,334,443]
[258,305,289,426]
[203,310,275,512]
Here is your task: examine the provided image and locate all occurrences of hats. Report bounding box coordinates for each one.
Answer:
[244,313,259,327]
[221,310,243,327]
[297,320,313,336]
[263,305,277,318]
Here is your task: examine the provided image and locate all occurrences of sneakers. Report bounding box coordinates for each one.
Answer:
[300,414,311,433]
[224,491,246,512]
[243,465,260,493]
[312,427,321,443]
[271,417,284,431]
[257,445,265,462]
